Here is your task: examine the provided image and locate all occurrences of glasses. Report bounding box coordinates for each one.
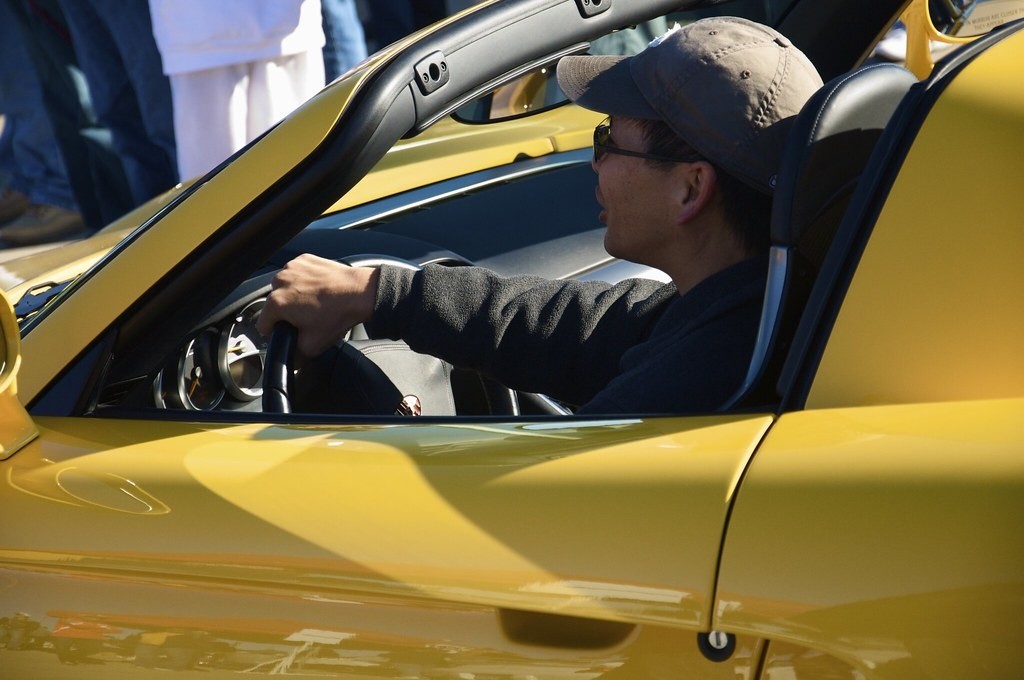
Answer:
[593,118,702,168]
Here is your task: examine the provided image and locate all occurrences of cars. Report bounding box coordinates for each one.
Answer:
[0,1,1024,680]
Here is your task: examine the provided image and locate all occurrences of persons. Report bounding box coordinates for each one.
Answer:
[256,14,826,415]
[0,0,370,243]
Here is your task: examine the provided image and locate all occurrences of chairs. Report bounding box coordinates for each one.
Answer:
[713,63,919,415]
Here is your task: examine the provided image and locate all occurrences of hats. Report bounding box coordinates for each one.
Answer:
[556,15,825,191]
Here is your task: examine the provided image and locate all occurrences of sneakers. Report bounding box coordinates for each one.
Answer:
[0,191,81,240]
